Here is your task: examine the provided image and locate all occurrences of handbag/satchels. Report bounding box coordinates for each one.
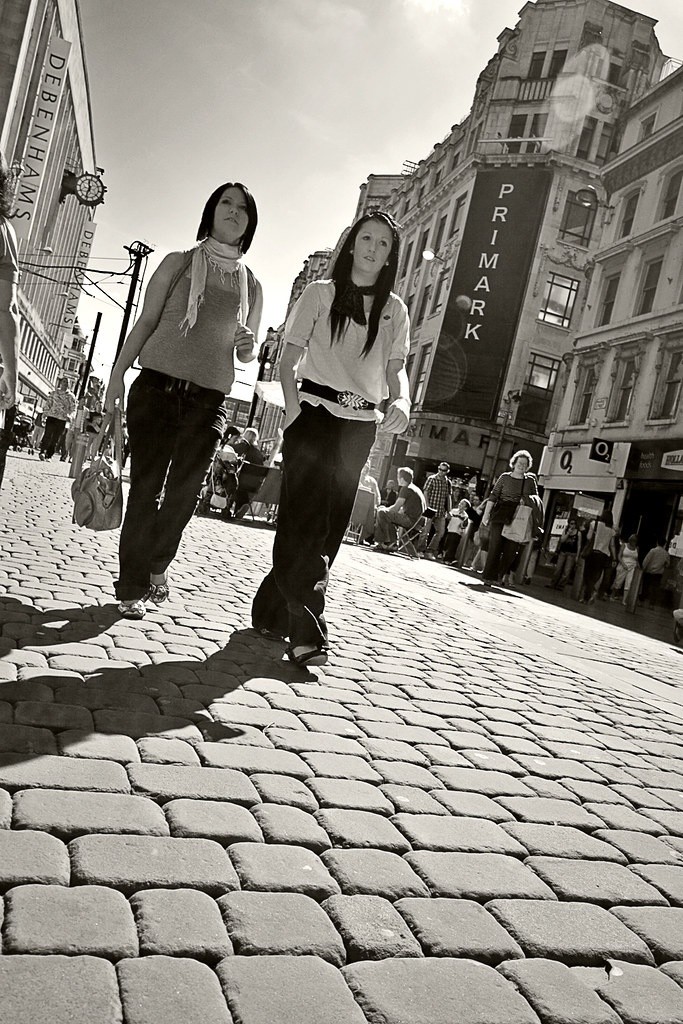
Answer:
[490,499,518,525]
[501,500,532,543]
[210,494,226,509]
[70,407,125,533]
[445,481,451,512]
[581,521,598,558]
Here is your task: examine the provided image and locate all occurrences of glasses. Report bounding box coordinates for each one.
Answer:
[571,523,576,525]
[438,467,447,471]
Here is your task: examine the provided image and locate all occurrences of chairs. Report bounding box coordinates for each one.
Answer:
[390,508,437,560]
[343,488,375,547]
[210,451,282,526]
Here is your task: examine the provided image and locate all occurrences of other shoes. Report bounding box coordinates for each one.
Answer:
[424,553,435,560]
[374,542,389,553]
[46,459,50,462]
[118,601,146,619]
[40,454,45,461]
[673,621,680,643]
[260,629,281,641]
[418,552,424,558]
[388,544,397,552]
[147,571,169,603]
[286,644,327,666]
[235,503,250,518]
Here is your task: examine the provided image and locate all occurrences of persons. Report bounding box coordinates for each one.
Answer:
[637,535,671,609]
[481,451,539,586]
[104,184,262,617]
[611,533,640,609]
[0,151,18,415]
[579,510,616,605]
[194,425,285,526]
[348,458,543,591]
[251,209,412,665]
[544,515,624,604]
[9,375,130,473]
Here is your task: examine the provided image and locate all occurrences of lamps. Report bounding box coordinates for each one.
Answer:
[503,389,522,405]
[422,248,445,263]
[575,185,614,211]
[35,247,53,255]
[55,292,69,299]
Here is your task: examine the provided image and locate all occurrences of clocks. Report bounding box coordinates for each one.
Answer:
[60,169,108,208]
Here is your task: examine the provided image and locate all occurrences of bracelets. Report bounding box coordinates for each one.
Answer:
[393,395,412,407]
[612,559,617,562]
[567,532,570,535]
